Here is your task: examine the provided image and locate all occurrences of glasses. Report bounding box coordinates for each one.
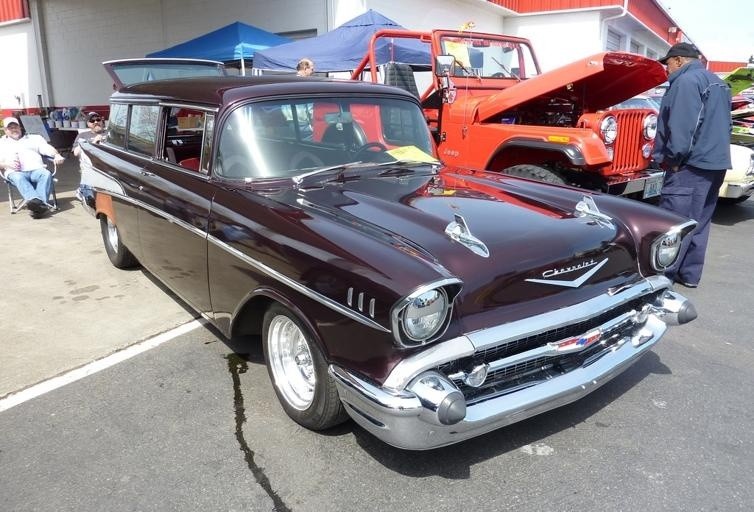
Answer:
[89,119,101,123]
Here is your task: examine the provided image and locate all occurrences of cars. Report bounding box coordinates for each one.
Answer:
[76,54,698,452]
[608,66,754,205]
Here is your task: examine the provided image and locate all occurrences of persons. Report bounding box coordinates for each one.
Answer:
[281,57,314,139]
[71,111,108,201]
[0,117,65,219]
[650,43,734,289]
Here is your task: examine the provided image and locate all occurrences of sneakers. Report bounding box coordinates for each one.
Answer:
[26,197,51,218]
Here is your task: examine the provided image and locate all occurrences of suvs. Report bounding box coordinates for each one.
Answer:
[310,28,665,203]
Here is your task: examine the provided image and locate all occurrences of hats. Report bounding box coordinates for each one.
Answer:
[86,112,100,121]
[658,42,698,64]
[3,117,19,128]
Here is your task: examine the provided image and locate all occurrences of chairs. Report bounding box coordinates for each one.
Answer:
[0,160,60,216]
[381,63,440,143]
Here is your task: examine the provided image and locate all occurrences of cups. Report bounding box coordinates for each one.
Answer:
[46,120,85,129]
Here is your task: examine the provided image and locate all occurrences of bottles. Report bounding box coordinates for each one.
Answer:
[14,153,20,171]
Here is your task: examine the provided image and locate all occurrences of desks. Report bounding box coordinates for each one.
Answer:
[52,126,107,160]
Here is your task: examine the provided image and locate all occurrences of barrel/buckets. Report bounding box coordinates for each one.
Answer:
[48,120,87,128]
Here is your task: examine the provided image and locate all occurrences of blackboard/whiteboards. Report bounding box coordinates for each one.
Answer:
[20,115,51,142]
